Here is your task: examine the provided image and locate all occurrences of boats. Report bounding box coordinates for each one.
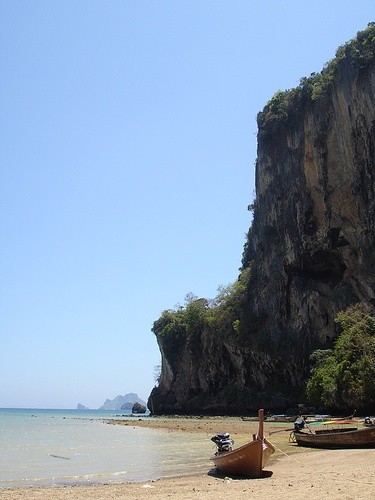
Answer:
[209,408,275,478]
[293,422,375,450]
[241,412,374,431]
